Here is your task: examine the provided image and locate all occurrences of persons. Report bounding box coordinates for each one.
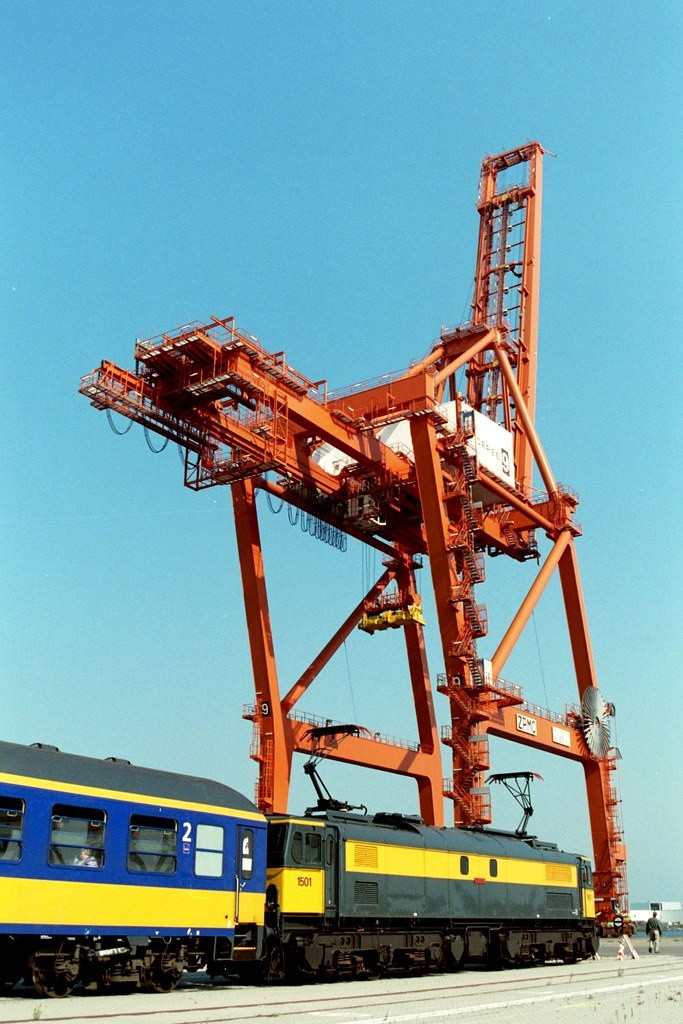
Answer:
[646,912,662,954]
[72,848,98,868]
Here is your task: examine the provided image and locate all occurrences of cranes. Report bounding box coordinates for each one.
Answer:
[78,140,639,941]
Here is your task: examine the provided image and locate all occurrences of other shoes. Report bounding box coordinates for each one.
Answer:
[649,947,652,953]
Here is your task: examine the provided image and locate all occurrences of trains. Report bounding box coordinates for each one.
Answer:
[0,736,605,1001]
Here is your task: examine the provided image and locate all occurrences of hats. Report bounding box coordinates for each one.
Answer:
[81,849,93,855]
